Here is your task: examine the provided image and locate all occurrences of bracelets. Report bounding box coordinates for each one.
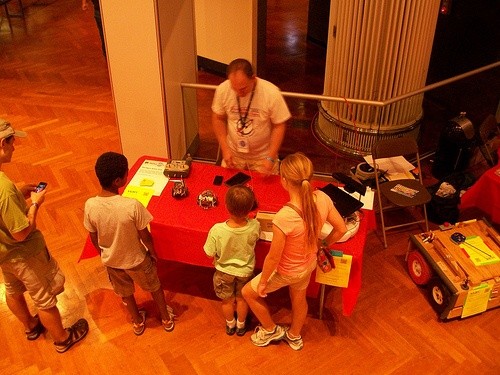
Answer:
[265,157,275,162]
[32,203,40,208]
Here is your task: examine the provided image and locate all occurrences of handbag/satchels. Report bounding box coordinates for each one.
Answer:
[317,239,336,274]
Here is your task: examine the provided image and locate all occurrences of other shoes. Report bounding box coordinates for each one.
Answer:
[132,306,145,335]
[226,311,237,336]
[162,305,174,331]
[236,313,252,336]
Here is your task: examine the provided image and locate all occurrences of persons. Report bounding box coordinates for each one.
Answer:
[203,187,261,335]
[210,59,291,179]
[241,152,346,351]
[83,152,174,335]
[0,117,89,352]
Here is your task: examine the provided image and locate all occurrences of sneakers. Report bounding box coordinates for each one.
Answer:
[283,332,304,350]
[251,325,285,347]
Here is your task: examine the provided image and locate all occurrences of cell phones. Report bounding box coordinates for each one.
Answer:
[214,176,223,185]
[226,172,251,188]
[34,182,47,193]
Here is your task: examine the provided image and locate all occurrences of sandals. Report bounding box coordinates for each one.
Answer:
[24,313,43,340]
[54,318,89,353]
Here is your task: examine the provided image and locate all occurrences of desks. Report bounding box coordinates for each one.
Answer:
[456,162,500,226]
[78,155,378,318]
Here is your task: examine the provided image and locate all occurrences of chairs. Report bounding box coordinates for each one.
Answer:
[370,136,431,248]
[480,114,500,167]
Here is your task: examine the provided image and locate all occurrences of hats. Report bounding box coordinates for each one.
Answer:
[0,118,27,140]
